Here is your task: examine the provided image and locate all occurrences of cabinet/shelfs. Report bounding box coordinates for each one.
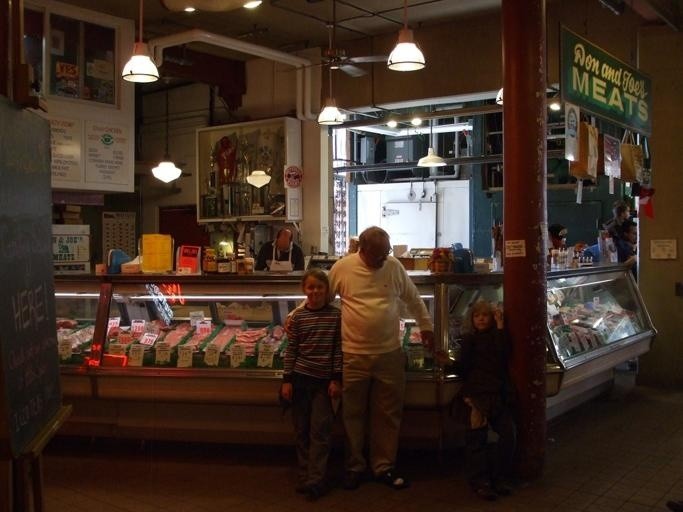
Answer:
[194,114,303,223]
[446,263,660,450]
[52,268,447,453]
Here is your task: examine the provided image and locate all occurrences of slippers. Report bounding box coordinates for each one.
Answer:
[375,471,410,489]
[343,471,360,489]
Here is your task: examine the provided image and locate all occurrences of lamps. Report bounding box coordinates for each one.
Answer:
[496,0,507,106]
[316,0,348,127]
[242,0,264,12]
[148,73,183,182]
[415,1,446,168]
[246,0,274,188]
[384,0,427,72]
[551,0,563,114]
[411,117,424,128]
[388,121,400,127]
[121,0,159,84]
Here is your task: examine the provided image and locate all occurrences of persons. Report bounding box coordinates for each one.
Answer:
[258,232,305,274]
[280,267,344,498]
[615,221,638,279]
[449,303,511,432]
[286,226,435,489]
[606,204,631,238]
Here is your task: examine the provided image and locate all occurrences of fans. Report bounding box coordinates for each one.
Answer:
[277,0,389,80]
